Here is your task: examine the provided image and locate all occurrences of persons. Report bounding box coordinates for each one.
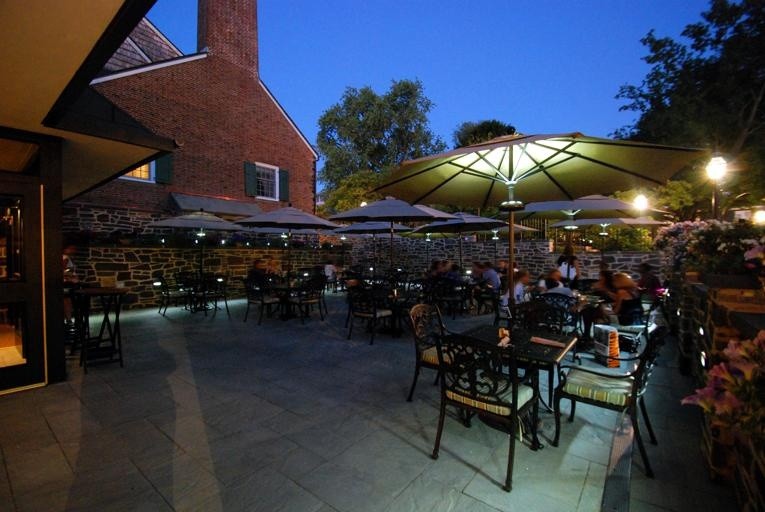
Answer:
[62,243,81,325]
[244,258,347,314]
[428,245,662,342]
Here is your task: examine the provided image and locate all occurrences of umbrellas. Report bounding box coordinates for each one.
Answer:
[480,220,541,233]
[498,192,671,287]
[232,201,339,285]
[143,208,241,316]
[333,221,413,271]
[406,210,509,273]
[362,125,714,330]
[550,215,673,273]
[240,226,322,273]
[326,195,462,276]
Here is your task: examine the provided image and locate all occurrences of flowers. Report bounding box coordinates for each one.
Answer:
[651,219,765,440]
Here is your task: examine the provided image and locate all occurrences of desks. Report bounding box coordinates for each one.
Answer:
[64,286,129,372]
